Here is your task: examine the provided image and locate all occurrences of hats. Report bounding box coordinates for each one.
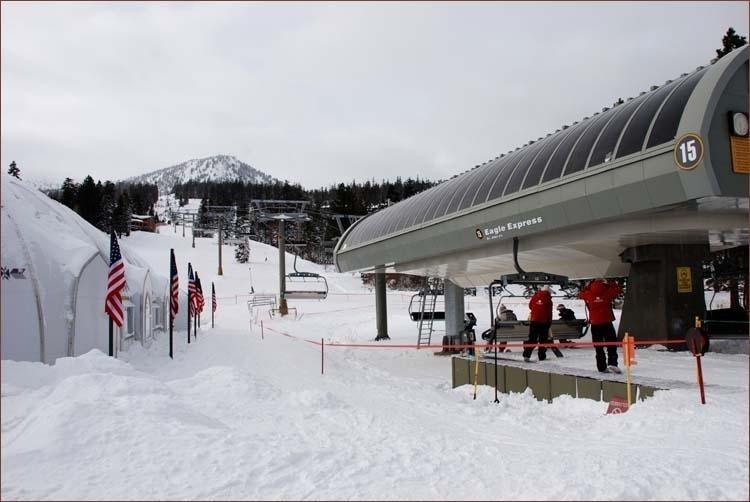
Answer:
[557,304,565,310]
[540,285,549,291]
[499,304,506,313]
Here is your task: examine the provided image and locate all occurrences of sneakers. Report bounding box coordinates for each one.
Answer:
[608,364,621,374]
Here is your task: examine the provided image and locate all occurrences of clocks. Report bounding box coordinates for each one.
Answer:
[726,108,750,138]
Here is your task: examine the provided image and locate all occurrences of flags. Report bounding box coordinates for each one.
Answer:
[211,284,218,313]
[105,231,128,327]
[194,274,201,315]
[198,278,205,312]
[189,264,198,317]
[169,251,181,325]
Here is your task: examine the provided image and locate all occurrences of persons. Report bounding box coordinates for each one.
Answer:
[575,278,623,374]
[522,309,533,349]
[556,303,578,351]
[523,284,555,363]
[484,303,517,356]
